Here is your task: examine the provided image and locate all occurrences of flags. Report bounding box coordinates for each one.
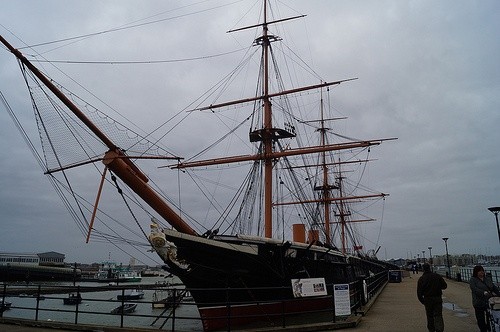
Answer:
[354,245,362,250]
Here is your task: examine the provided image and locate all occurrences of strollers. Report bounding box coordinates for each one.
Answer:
[482,292,500,332]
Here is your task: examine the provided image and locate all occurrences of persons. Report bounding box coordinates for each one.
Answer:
[417,263,447,332]
[412,264,418,274]
[470,265,494,332]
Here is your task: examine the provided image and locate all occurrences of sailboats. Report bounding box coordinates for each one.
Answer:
[0,0,400,332]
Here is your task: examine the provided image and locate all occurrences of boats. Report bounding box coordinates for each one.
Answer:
[110,303,138,314]
[152,280,194,309]
[62,292,83,306]
[116,288,144,300]
[0,298,12,317]
[91,257,142,283]
[18,292,46,301]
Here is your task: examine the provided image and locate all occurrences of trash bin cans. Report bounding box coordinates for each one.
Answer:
[446,271,448,277]
[457,273,461,280]
[486,272,492,280]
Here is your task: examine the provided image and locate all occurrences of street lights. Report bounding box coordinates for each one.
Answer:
[428,247,433,273]
[417,254,420,263]
[422,251,425,270]
[488,207,500,241]
[442,237,451,279]
